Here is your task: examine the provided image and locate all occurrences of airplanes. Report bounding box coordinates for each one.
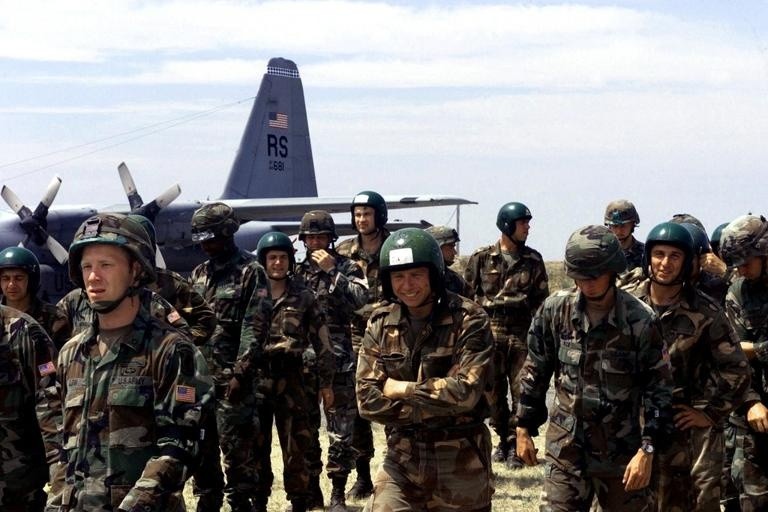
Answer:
[0,58,481,276]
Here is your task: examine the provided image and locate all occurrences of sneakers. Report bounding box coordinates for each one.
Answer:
[494,436,522,469]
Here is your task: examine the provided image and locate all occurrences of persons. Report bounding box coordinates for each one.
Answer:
[0,190,768,511]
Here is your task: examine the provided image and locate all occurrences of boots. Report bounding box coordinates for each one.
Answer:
[330,477,347,512]
[346,460,373,498]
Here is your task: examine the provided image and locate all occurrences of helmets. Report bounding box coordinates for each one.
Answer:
[257,191,460,297]
[564,200,768,281]
[191,203,239,238]
[68,213,157,287]
[0,247,40,293]
[497,202,531,236]
